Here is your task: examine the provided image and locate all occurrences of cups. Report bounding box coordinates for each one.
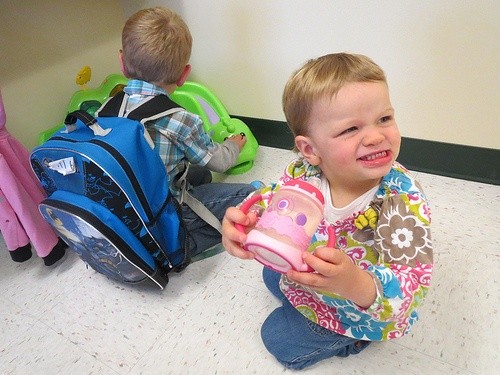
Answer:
[236,176,336,274]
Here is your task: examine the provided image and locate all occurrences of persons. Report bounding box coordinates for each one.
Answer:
[95,7,258,258]
[222,53,433,371]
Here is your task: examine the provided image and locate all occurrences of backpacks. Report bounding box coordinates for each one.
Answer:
[28,91,222,291]
